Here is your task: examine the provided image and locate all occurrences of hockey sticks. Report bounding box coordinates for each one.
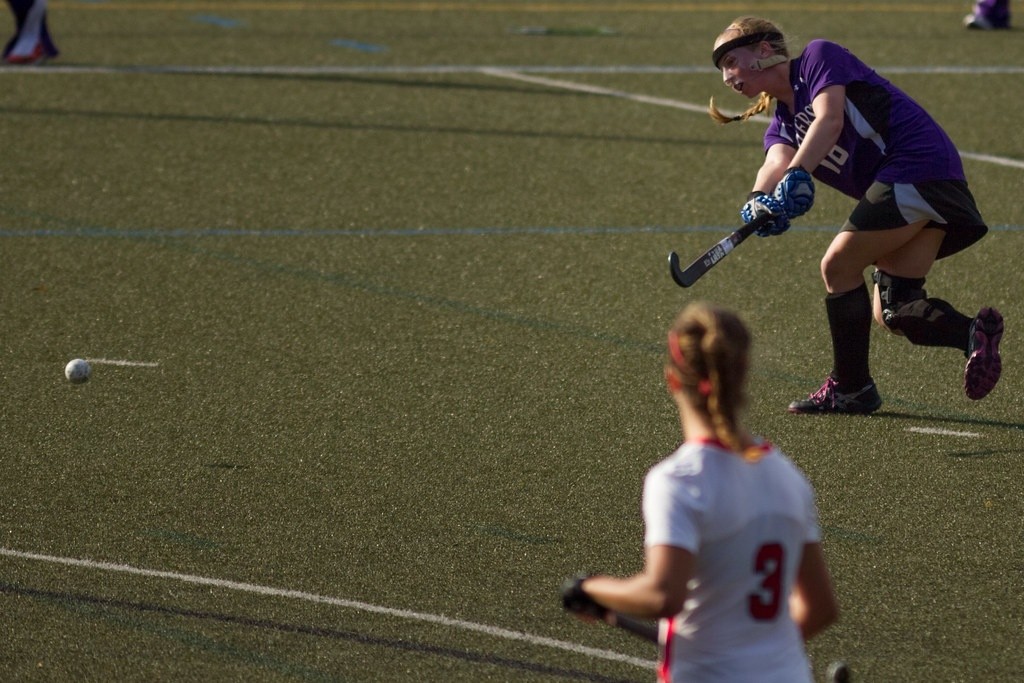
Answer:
[668,213,771,289]
[569,580,849,683]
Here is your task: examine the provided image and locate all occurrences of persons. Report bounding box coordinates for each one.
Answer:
[962,0,1013,30]
[560,299,841,683]
[0,0,60,66]
[708,16,1005,416]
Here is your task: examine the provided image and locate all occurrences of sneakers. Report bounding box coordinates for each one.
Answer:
[964,307,1004,400]
[788,373,882,414]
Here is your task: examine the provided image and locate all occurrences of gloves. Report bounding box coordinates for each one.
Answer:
[771,165,815,219]
[563,579,609,621]
[740,192,791,237]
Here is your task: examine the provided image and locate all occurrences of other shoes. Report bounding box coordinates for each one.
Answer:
[0,34,58,64]
[963,14,1010,30]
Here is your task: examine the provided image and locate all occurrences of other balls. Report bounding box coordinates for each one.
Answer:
[64,359,92,382]
